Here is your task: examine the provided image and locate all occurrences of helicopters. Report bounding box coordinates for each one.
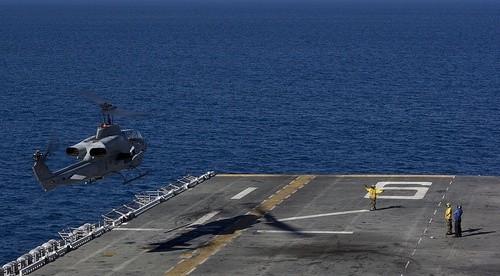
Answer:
[33,94,151,192]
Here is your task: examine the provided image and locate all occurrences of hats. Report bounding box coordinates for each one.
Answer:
[457,202,462,210]
[445,202,450,208]
[371,185,375,189]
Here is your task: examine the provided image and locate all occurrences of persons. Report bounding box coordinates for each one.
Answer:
[363,184,385,211]
[453,205,463,238]
[444,203,453,235]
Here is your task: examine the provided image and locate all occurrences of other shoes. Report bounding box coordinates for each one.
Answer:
[446,230,451,234]
[370,207,376,211]
[453,234,459,238]
[459,233,463,237]
